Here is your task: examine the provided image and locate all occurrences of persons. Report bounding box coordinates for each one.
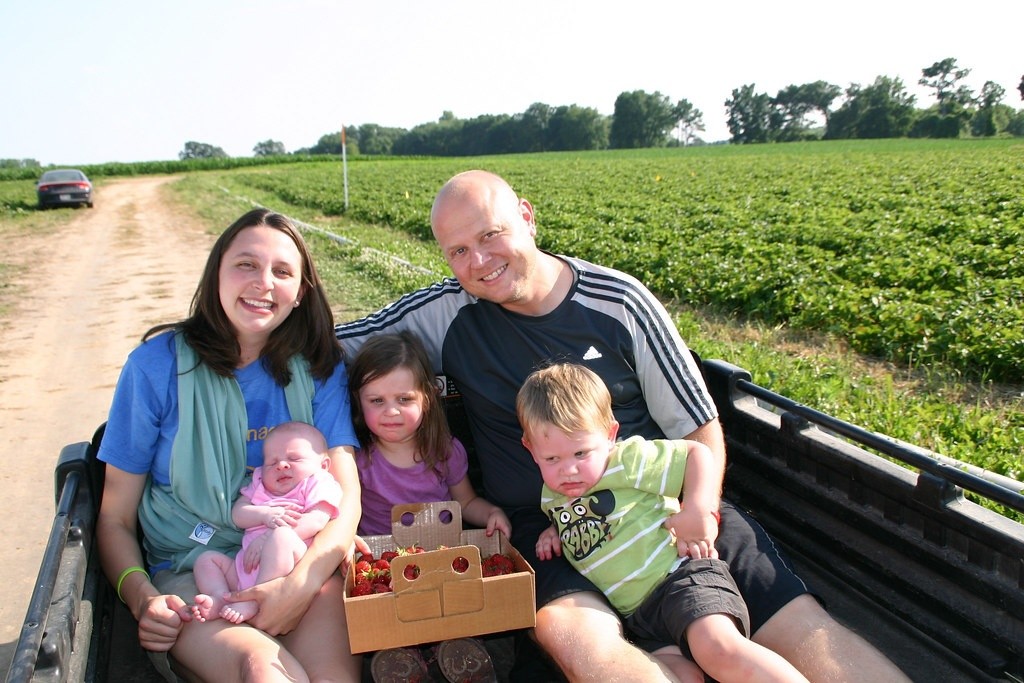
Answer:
[95,170,919,683]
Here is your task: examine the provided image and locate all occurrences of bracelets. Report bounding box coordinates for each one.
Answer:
[681,500,722,525]
[116,566,150,605]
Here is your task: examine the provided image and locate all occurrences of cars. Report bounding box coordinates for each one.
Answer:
[33,168,93,211]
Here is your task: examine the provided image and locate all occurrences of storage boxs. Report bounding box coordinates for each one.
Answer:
[341,501,537,655]
